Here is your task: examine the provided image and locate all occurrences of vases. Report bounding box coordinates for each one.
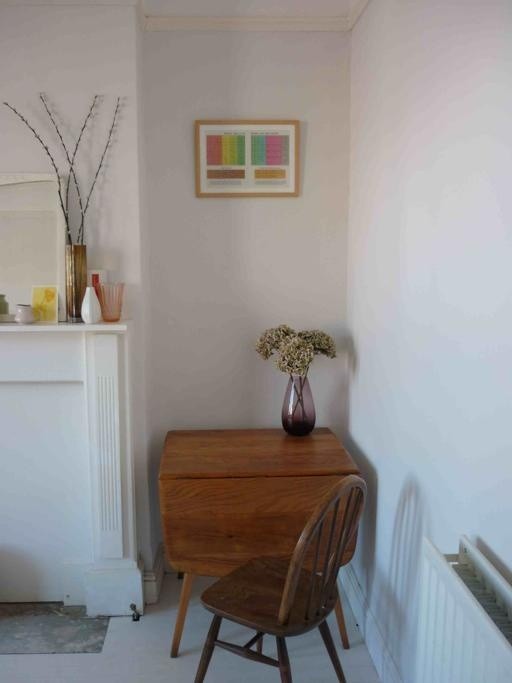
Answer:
[281,372,318,436]
[66,244,87,322]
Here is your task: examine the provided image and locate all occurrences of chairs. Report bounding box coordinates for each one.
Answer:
[191,474,369,682]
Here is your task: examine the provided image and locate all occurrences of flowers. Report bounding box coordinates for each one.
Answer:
[254,322,338,422]
[2,89,124,245]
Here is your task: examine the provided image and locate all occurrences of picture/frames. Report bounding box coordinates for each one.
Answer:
[193,117,303,198]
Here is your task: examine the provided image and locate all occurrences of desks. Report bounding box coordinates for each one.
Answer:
[157,430,366,663]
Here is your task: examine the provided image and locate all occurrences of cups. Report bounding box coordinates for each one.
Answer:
[14,303,41,324]
[98,283,124,322]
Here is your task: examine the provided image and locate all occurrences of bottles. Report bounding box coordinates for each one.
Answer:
[0,293,9,322]
[82,273,102,322]
